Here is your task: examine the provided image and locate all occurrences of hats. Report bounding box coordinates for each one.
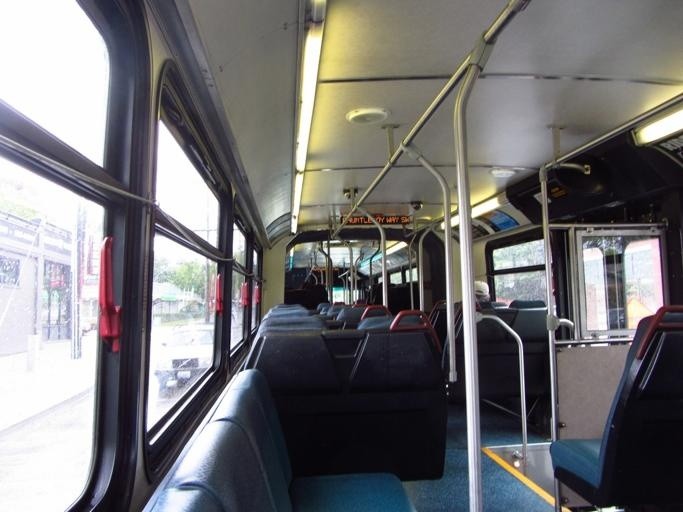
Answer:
[473,281,489,295]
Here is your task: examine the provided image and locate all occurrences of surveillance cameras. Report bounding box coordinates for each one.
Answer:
[412,202,424,211]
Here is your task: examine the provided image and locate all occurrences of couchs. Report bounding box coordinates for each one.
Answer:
[140,369,417,512]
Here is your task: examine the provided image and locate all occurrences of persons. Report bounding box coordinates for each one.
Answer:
[474,280,494,309]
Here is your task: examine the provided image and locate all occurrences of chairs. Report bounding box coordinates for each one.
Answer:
[550,303,682,508]
[245,298,549,480]
